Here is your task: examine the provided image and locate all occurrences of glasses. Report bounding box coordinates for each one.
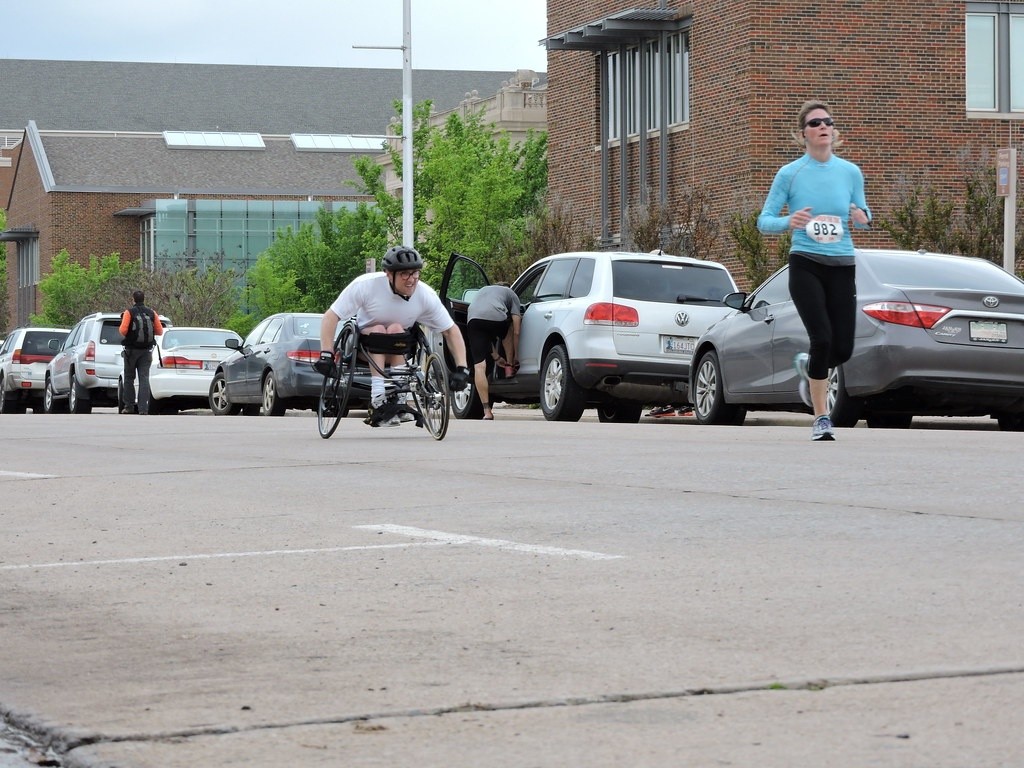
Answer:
[804,117,834,127]
[389,270,421,280]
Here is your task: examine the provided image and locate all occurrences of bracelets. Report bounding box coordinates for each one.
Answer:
[495,356,501,361]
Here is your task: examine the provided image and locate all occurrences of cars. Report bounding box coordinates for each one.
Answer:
[206,312,340,417]
[684,246,1024,442]
[115,325,244,414]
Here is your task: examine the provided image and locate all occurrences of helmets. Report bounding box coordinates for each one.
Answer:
[381,246,423,271]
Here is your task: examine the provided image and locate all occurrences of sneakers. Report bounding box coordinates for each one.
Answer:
[371,394,400,425]
[811,415,835,441]
[388,392,416,422]
[795,352,813,406]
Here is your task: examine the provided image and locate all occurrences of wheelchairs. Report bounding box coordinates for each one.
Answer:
[312,316,452,442]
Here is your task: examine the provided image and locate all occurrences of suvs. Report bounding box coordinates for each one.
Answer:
[0,326,74,414]
[43,309,175,415]
[436,251,748,424]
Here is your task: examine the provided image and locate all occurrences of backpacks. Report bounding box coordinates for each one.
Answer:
[130,307,154,343]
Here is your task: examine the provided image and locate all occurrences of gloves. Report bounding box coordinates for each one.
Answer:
[314,351,336,377]
[449,366,471,391]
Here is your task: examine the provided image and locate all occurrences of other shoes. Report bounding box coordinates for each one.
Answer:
[505,365,516,378]
[483,408,494,420]
[121,406,134,414]
[138,408,148,414]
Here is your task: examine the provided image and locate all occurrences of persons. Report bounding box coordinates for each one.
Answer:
[758,101,872,441]
[314,247,470,427]
[467,282,521,420]
[119,291,163,414]
